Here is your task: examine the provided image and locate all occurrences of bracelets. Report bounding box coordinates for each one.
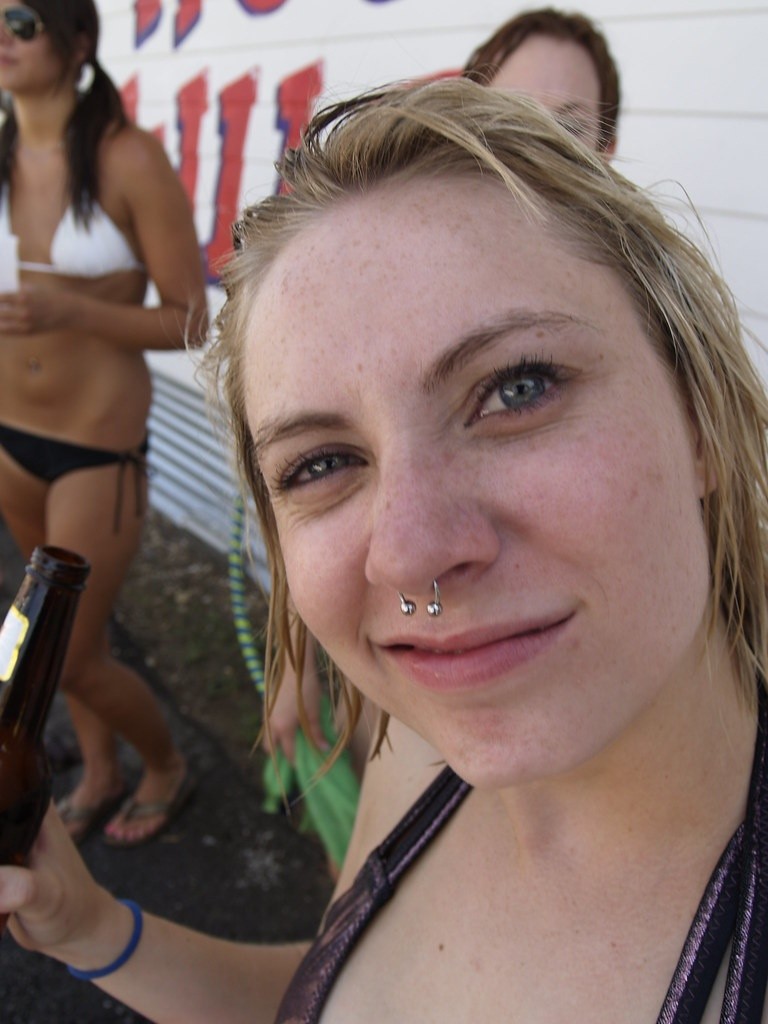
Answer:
[67,898,142,980]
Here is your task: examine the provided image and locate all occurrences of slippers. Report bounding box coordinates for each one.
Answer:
[53,785,128,852]
[101,764,200,849]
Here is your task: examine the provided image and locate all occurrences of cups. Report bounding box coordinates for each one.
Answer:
[0,233,20,293]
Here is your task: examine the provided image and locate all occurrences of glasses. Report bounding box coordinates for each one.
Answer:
[0,10,51,41]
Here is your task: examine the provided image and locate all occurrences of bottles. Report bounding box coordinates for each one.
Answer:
[0,545,93,939]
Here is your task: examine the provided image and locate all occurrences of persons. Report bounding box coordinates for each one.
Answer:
[0,76,768,1024]
[262,6,623,883]
[1,2,209,843]
